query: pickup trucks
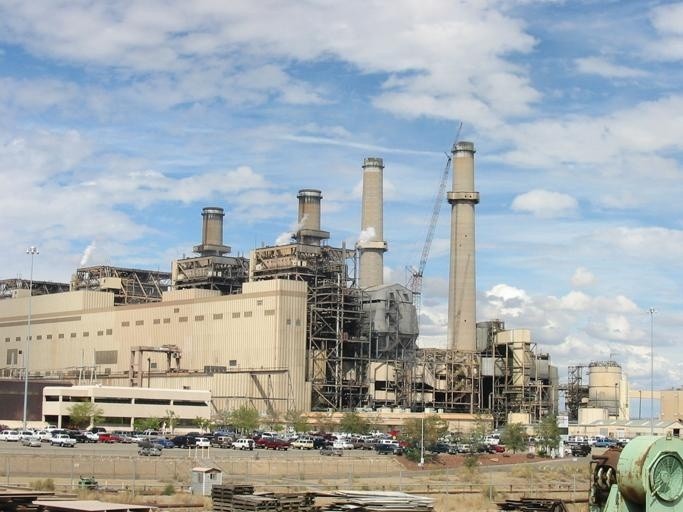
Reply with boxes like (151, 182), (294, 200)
(49, 434), (77, 449)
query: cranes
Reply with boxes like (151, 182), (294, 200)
(406, 119), (464, 349)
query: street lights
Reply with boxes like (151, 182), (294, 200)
(637, 389), (641, 420)
(417, 362), (427, 467)
(146, 357), (151, 388)
(645, 306), (659, 437)
(614, 382), (619, 420)
(20, 245), (40, 427)
(539, 380), (543, 422)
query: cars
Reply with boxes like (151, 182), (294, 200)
(0, 429), (17, 441)
(37, 430), (51, 440)
(530, 435), (631, 457)
(44, 424), (505, 457)
(20, 436), (41, 447)
(18, 430), (36, 438)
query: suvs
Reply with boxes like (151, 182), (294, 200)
(231, 438), (256, 451)
(289, 440), (314, 450)
